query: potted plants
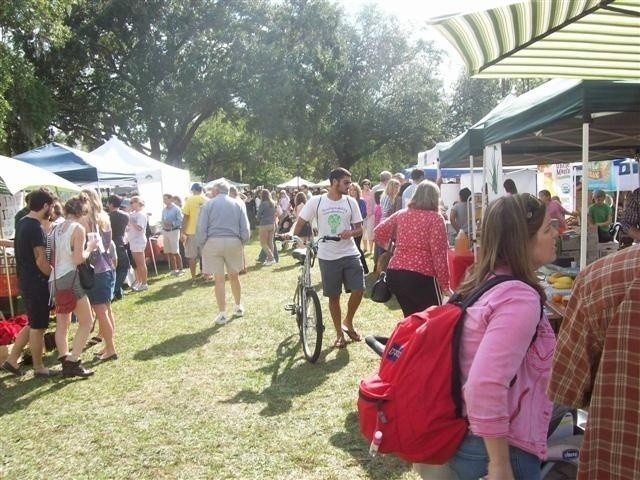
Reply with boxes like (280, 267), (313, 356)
(44, 315), (56, 352)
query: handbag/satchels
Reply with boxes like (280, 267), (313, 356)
(55, 287), (76, 313)
(369, 270), (393, 305)
(79, 262), (97, 289)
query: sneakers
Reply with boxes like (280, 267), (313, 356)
(132, 269), (212, 293)
(232, 302), (244, 316)
(1, 359), (26, 377)
(214, 310), (228, 325)
(31, 369), (58, 380)
(262, 258), (276, 266)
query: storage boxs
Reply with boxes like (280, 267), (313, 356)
(560, 234), (619, 265)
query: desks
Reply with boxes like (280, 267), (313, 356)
(447, 249), (474, 290)
(146, 239), (166, 277)
(541, 285), (569, 328)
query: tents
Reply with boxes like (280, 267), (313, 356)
(276, 174), (331, 187)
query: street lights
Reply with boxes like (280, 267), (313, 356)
(294, 153), (302, 191)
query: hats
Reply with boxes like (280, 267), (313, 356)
(190, 182), (202, 192)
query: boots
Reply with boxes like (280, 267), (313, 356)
(58, 353), (94, 379)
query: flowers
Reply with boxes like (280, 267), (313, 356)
(0, 315), (29, 345)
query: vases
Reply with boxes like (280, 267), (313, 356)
(0, 344), (23, 366)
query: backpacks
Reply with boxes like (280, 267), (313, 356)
(354, 271), (545, 467)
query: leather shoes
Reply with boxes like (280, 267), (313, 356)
(92, 349), (119, 363)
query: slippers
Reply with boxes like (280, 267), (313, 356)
(334, 333), (346, 348)
(342, 323), (362, 341)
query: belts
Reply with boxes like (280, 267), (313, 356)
(162, 225), (180, 232)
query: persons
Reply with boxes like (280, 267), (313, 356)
(4, 188), (118, 377)
(451, 178), (640, 247)
(243, 185), (299, 267)
(362, 169), (453, 319)
(159, 181), (246, 281)
(295, 167), (367, 346)
(105, 194), (148, 298)
(198, 180), (249, 324)
(543, 244), (639, 478)
(450, 194), (557, 480)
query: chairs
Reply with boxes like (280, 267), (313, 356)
(539, 432), (584, 480)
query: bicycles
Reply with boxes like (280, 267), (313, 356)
(270, 232), (343, 362)
